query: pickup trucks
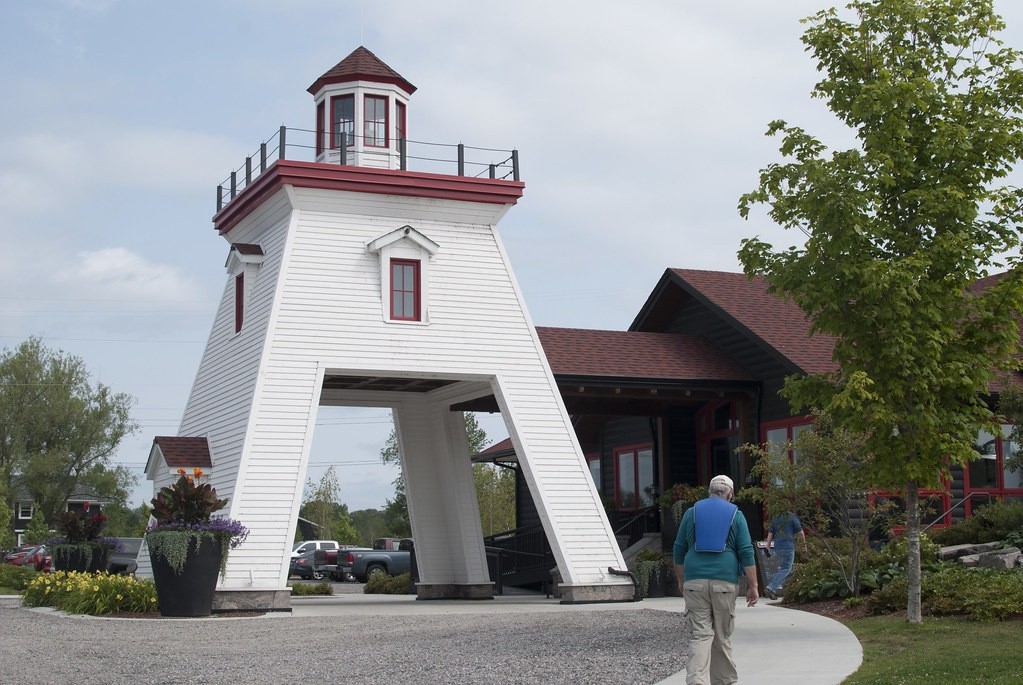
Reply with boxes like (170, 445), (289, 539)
(336, 537), (414, 584)
(315, 537), (402, 583)
(287, 538), (375, 579)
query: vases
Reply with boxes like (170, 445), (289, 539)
(53, 548), (110, 574)
(147, 532), (231, 617)
(648, 565), (668, 598)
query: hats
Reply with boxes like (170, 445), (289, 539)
(710, 475), (735, 502)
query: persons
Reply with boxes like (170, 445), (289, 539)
(672, 475), (759, 685)
(765, 501), (806, 599)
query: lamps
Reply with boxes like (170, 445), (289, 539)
(650, 483), (659, 506)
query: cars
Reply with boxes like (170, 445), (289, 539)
(2, 544), (50, 571)
(291, 549), (341, 581)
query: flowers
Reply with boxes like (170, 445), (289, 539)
(45, 502), (126, 571)
(145, 465), (250, 577)
(657, 482), (763, 529)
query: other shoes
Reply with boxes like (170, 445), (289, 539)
(763, 587), (777, 600)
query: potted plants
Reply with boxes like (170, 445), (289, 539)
(635, 549), (666, 598)
